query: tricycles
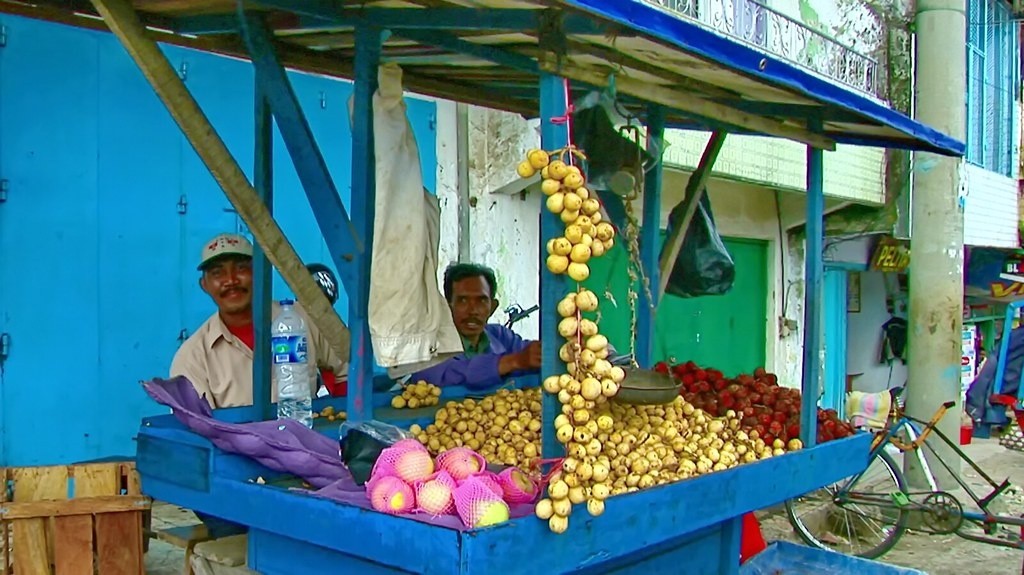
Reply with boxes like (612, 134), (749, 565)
(785, 380), (1024, 562)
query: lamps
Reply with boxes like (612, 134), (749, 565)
(610, 97), (645, 201)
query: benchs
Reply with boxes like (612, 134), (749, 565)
(157, 524), (212, 575)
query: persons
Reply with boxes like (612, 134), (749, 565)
(403, 262), (542, 391)
(169, 234), (348, 538)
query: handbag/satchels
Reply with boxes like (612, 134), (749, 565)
(662, 171), (735, 298)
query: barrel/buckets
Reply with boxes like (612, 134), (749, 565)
(961, 427), (973, 445)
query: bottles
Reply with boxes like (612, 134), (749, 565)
(271, 298), (313, 432)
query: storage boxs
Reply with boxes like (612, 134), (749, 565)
(0, 461), (152, 575)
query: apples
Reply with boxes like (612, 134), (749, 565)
(372, 448), (534, 527)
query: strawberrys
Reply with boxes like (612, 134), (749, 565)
(648, 360), (855, 447)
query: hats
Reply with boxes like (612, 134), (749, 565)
(196, 232), (254, 270)
